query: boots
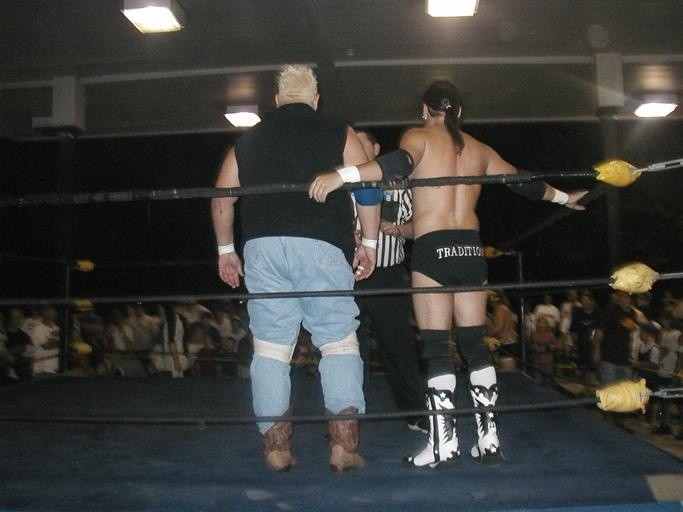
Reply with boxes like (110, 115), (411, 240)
(323, 406), (368, 473)
(403, 387), (462, 467)
(259, 405), (298, 473)
(468, 381), (506, 465)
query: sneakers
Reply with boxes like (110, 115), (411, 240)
(407, 415), (430, 434)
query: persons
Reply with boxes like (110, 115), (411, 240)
(3, 301), (321, 382)
(452, 286), (683, 443)
(208, 65), (385, 474)
(350, 129), (432, 434)
(307, 79), (590, 468)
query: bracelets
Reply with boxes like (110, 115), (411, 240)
(551, 188), (570, 205)
(360, 236), (378, 249)
(337, 166), (362, 185)
(217, 241), (236, 257)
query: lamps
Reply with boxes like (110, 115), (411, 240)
(425, 0), (480, 19)
(223, 105), (264, 129)
(123, 0), (187, 36)
(631, 94), (679, 119)
